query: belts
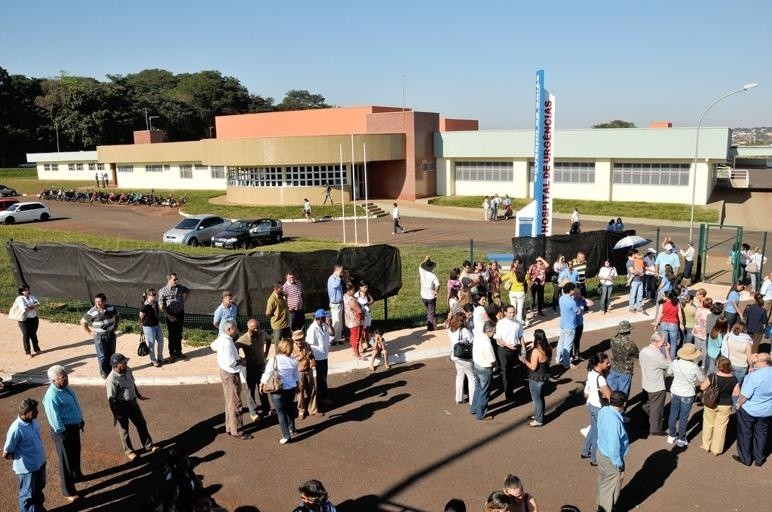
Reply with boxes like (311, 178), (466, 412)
(333, 302), (342, 304)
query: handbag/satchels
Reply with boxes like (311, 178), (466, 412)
(598, 385), (612, 406)
(597, 284), (603, 294)
(138, 340), (148, 356)
(455, 342), (473, 358)
(745, 262), (758, 273)
(8, 304), (27, 322)
(262, 374), (282, 393)
(504, 279), (513, 290)
(701, 385), (719, 409)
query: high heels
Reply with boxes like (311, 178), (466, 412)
(457, 400), (466, 405)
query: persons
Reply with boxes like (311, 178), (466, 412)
(483, 192), (513, 221)
(138, 287), (173, 366)
(606, 219), (616, 230)
(2, 397), (50, 512)
(158, 271), (191, 363)
(320, 182), (334, 206)
(94, 172), (110, 188)
(390, 202), (407, 235)
(10, 279), (48, 358)
(208, 263), (392, 446)
(42, 364), (92, 505)
(301, 198), (314, 224)
(417, 233), (772, 512)
(614, 217), (625, 231)
(79, 292), (121, 379)
(105, 352), (163, 461)
(235, 478), (339, 512)
(566, 205), (583, 235)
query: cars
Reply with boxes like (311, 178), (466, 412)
(0, 199), (51, 224)
(0, 185), (18, 197)
(162, 214), (232, 249)
(0, 198), (19, 211)
(209, 215), (283, 248)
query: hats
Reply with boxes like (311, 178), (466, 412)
(421, 258), (436, 271)
(314, 309), (331, 318)
(677, 343), (701, 360)
(648, 247), (657, 253)
(616, 321), (633, 333)
(111, 353), (130, 364)
(292, 330), (304, 340)
(665, 243), (673, 250)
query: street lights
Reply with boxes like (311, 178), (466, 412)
(688, 82), (759, 245)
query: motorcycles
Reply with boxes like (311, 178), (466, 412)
(39, 187), (177, 208)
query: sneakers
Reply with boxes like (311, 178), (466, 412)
(624, 416), (630, 423)
(226, 409), (325, 445)
(67, 494), (80, 501)
(485, 415), (493, 419)
(652, 431), (689, 447)
(128, 452), (137, 460)
(322, 398), (333, 404)
(529, 421), (542, 426)
(153, 354), (187, 366)
(151, 446), (161, 453)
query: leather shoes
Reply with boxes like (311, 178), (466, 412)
(732, 455), (742, 462)
